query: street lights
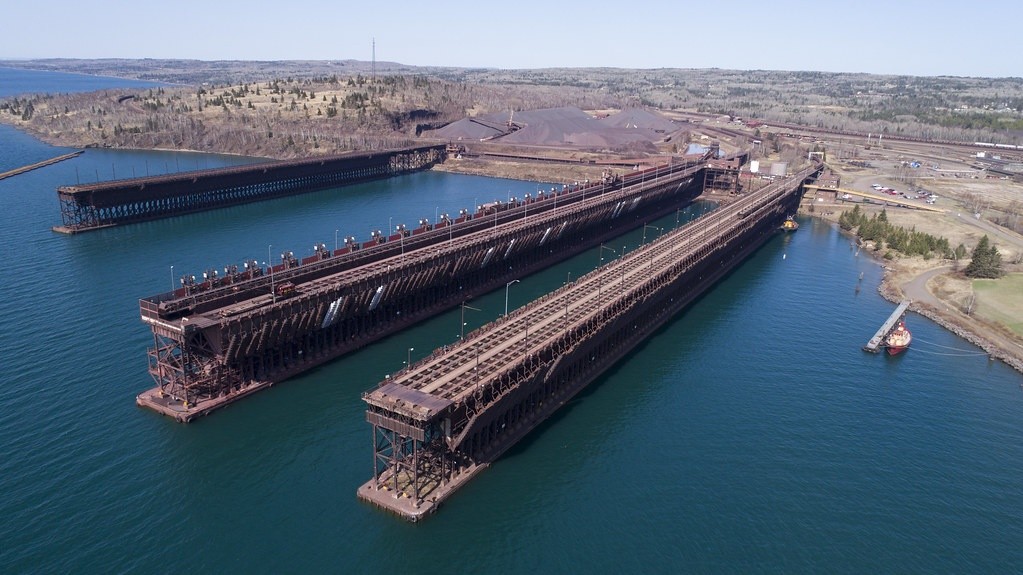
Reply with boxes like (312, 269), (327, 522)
(518, 198), (526, 224)
(389, 217), (393, 236)
(262, 262), (275, 305)
(408, 347), (414, 363)
(269, 245), (272, 266)
(171, 266), (174, 291)
(599, 242), (615, 268)
(677, 207), (686, 227)
(505, 279), (520, 316)
(548, 190), (556, 216)
(335, 229), (339, 250)
(435, 206), (439, 226)
(642, 222), (659, 246)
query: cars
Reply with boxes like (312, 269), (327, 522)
(871, 183), (938, 203)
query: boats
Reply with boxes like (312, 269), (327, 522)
(780, 215), (799, 232)
(885, 321), (913, 356)
